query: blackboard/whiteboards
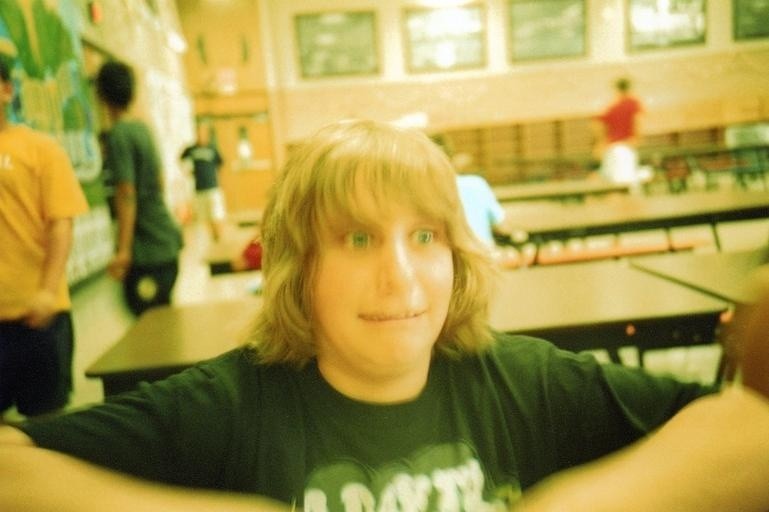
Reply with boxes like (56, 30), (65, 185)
(0, 0), (119, 296)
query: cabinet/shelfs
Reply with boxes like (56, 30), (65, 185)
(449, 111), (609, 180)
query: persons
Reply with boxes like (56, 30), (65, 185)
(429, 130), (508, 266)
(594, 79), (648, 179)
(0, 52), (92, 422)
(0, 117), (769, 511)
(179, 121), (227, 241)
(93, 62), (187, 315)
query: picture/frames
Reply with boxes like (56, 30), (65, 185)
(291, 1), (769, 82)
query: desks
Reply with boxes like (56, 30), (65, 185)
(472, 138), (768, 386)
(85, 210), (266, 402)
